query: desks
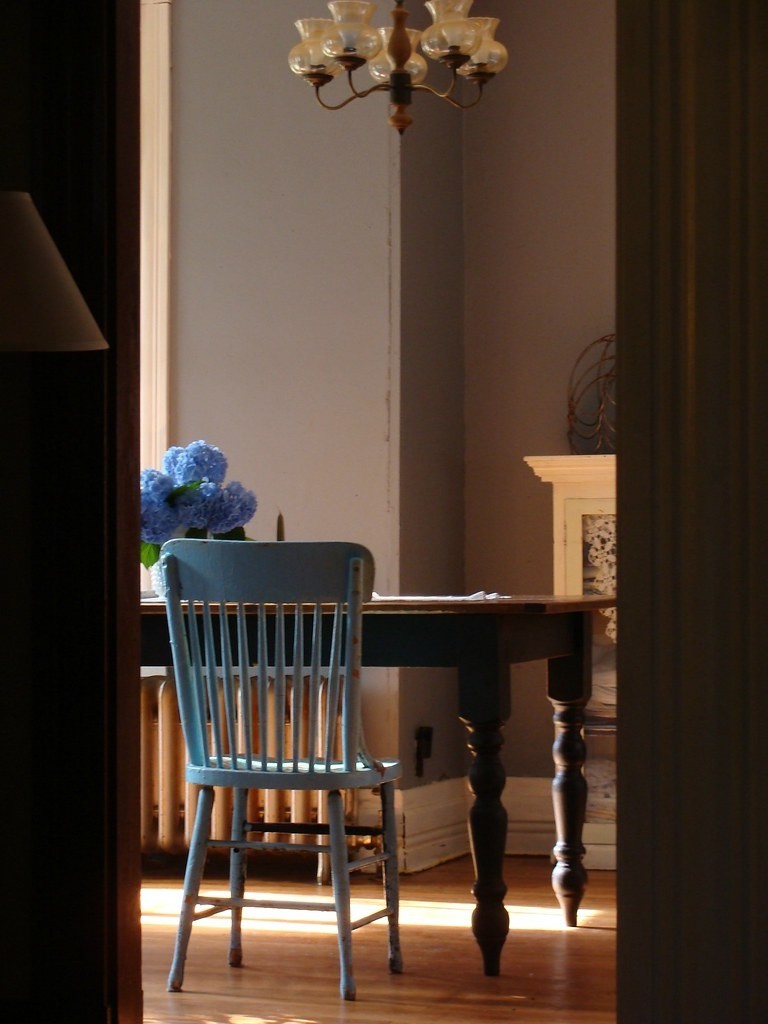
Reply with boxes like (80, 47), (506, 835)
(141, 596), (617, 975)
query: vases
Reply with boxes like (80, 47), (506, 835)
(150, 523), (214, 598)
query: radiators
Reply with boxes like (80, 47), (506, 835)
(141, 675), (352, 886)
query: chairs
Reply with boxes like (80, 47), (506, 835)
(159, 538), (402, 1000)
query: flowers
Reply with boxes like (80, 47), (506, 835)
(139, 440), (257, 568)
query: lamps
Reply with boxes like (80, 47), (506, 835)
(288, 0), (509, 135)
(1, 189), (109, 352)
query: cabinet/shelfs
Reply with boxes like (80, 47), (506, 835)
(523, 454), (616, 869)
